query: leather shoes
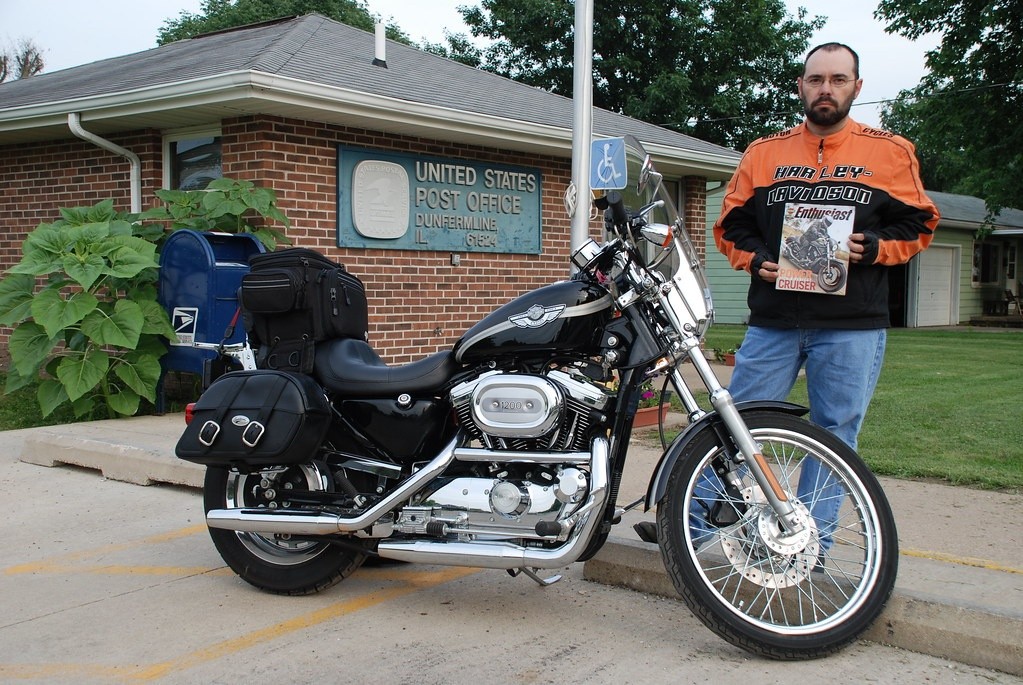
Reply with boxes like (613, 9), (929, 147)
(812, 555), (825, 574)
(633, 520), (699, 551)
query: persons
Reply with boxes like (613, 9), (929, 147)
(799, 216), (834, 257)
(633, 41), (940, 575)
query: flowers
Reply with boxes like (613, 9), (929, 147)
(610, 376), (663, 409)
(728, 344), (741, 354)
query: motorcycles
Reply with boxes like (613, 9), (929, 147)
(782, 229), (846, 293)
(174, 133), (900, 663)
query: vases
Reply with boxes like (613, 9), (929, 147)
(631, 402), (670, 429)
(725, 353), (736, 366)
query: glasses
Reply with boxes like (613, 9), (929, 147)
(803, 77), (855, 87)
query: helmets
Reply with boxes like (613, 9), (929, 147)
(822, 214), (833, 228)
